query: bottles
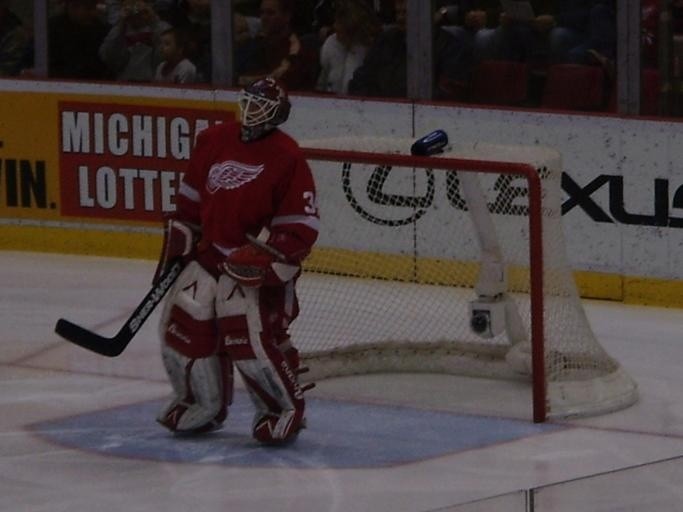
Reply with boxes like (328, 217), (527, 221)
(411, 129), (450, 155)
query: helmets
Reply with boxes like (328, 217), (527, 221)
(238, 77), (290, 143)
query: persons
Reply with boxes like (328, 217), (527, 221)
(0, 0), (683, 120)
(150, 75), (319, 441)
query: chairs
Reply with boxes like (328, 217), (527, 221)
(474, 58), (662, 115)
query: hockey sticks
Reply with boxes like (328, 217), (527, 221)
(54, 261), (184, 357)
